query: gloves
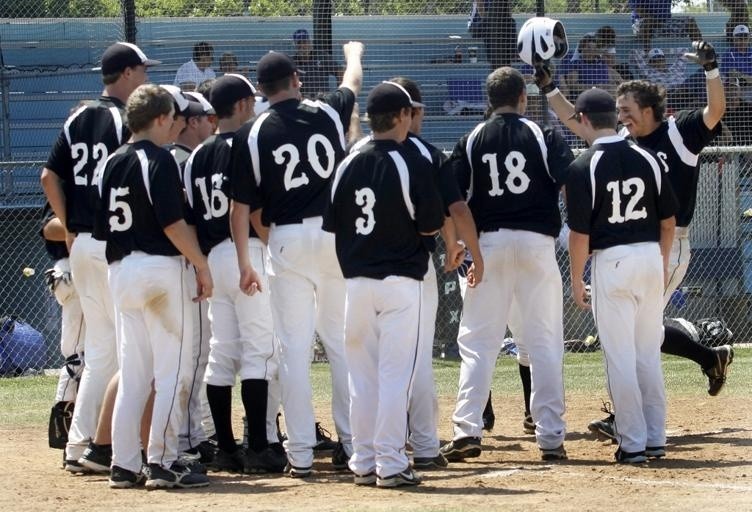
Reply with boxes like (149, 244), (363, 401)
(44, 269), (73, 291)
(684, 40), (718, 71)
(531, 61), (556, 94)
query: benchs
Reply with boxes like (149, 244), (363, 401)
(0, 12), (751, 205)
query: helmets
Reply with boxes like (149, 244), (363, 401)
(517, 16), (569, 65)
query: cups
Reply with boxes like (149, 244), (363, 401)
(741, 208), (752, 225)
(468, 47), (478, 63)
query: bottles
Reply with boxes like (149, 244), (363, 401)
(23, 267), (35, 277)
(631, 20), (640, 35)
(677, 286), (704, 298)
(455, 44), (462, 63)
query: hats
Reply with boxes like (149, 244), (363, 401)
(293, 30), (308, 42)
(647, 48), (664, 62)
(102, 41), (163, 74)
(257, 49), (307, 82)
(366, 80), (427, 113)
(568, 86), (616, 121)
(210, 73), (257, 102)
(159, 84), (216, 116)
(733, 24), (749, 36)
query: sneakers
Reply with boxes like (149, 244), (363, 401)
(615, 446), (665, 463)
(540, 445), (567, 460)
(481, 415), (494, 429)
(441, 439), (482, 461)
(705, 344), (734, 396)
(314, 421), (339, 451)
(413, 450), (449, 469)
(524, 412), (537, 435)
(588, 414), (615, 440)
(188, 433), (313, 477)
(333, 443), (422, 488)
(66, 440), (209, 488)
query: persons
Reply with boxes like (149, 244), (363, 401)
(40, 0), (751, 490)
